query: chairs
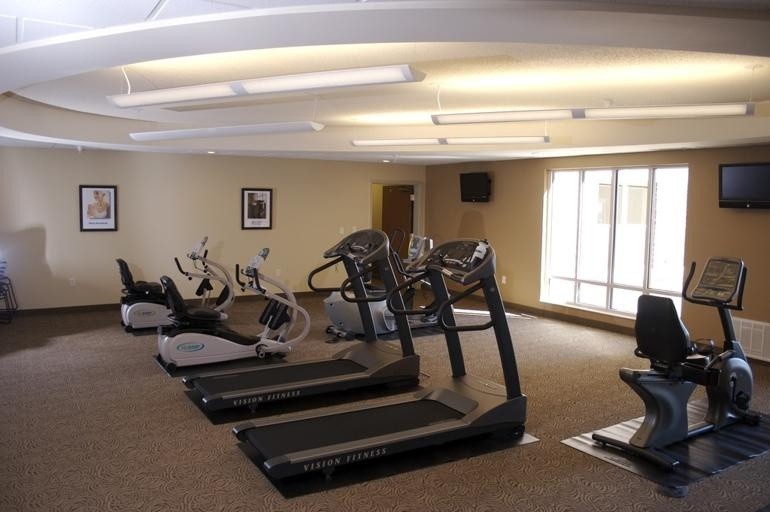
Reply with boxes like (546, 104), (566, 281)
(634, 295), (716, 380)
(160, 276), (221, 329)
(116, 258), (163, 296)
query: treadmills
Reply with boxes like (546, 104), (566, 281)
(182, 230), (420, 412)
(234, 239), (526, 478)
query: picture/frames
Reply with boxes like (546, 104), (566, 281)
(241, 187), (272, 231)
(79, 184), (117, 233)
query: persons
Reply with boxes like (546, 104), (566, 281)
(87, 191), (111, 219)
(248, 194), (259, 218)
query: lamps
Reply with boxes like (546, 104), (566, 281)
(344, 115), (551, 149)
(428, 66), (761, 126)
(103, 57), (421, 108)
(129, 96), (326, 144)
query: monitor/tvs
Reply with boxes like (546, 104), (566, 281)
(719, 162), (770, 209)
(460, 172), (489, 202)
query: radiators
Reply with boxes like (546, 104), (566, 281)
(728, 314), (770, 363)
